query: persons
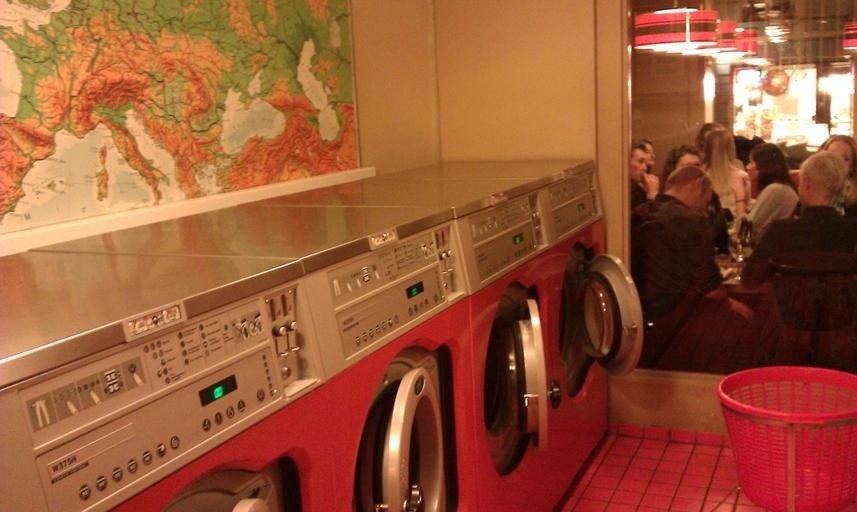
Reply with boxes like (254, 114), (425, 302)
(630, 123), (856, 327)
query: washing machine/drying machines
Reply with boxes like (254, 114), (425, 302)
(238, 179), (566, 512)
(0, 249), (337, 512)
(27, 205), (478, 512)
(361, 159), (645, 502)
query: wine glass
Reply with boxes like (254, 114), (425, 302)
(729, 231), (748, 283)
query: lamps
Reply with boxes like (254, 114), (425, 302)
(843, 21), (857, 50)
(633, 0), (759, 56)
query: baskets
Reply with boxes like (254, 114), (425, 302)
(716, 365), (857, 512)
